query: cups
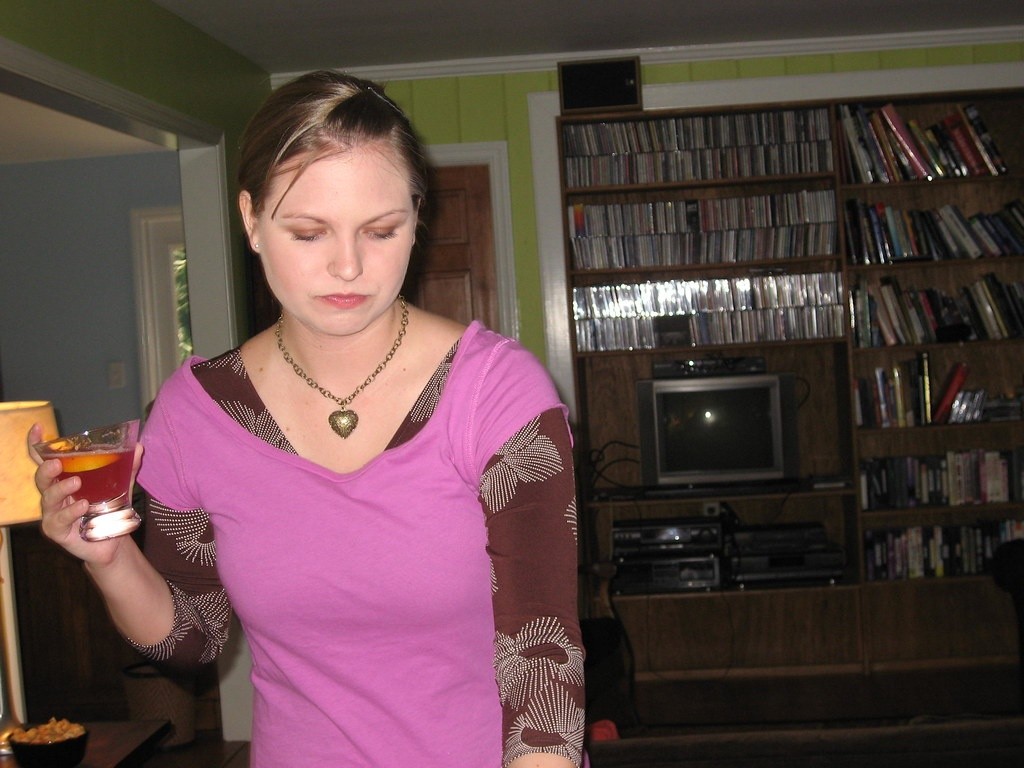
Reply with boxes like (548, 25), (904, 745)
(32, 417), (140, 540)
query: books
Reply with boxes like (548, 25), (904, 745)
(570, 273), (1024, 352)
(853, 349), (1024, 426)
(564, 103), (1024, 182)
(858, 440), (1024, 512)
(564, 191), (1024, 265)
(862, 515), (1024, 586)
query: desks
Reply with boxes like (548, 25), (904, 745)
(0, 719), (173, 768)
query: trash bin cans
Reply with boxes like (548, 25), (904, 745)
(119, 661), (198, 749)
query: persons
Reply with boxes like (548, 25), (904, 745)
(28, 69), (585, 768)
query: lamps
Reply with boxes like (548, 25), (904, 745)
(0, 400), (61, 726)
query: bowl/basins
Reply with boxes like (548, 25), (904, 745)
(7, 725), (89, 768)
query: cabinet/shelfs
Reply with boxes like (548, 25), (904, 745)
(551, 87), (1024, 768)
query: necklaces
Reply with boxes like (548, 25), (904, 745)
(275, 293), (409, 441)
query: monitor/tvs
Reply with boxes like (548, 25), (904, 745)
(637, 373), (786, 498)
